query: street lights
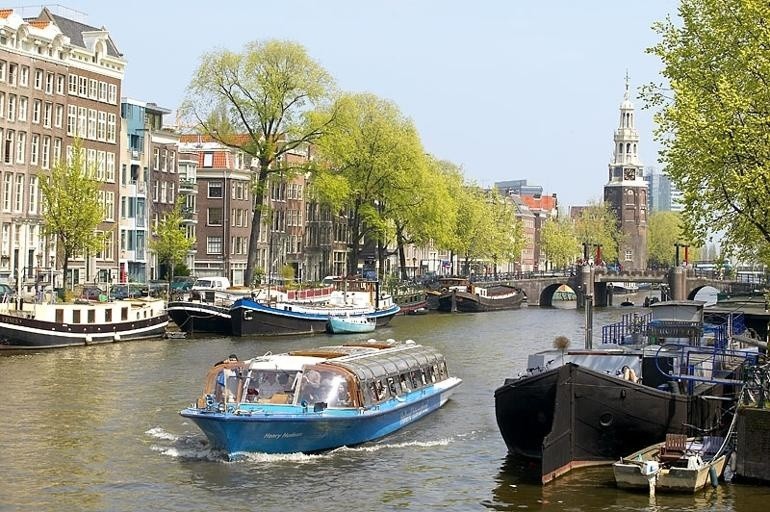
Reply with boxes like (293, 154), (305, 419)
(412, 257), (416, 280)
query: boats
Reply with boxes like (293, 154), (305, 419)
(1, 296), (169, 356)
(167, 276), (401, 336)
(439, 279), (524, 310)
(396, 294), (429, 314)
(179, 339), (462, 462)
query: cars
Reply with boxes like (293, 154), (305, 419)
(1, 285), (15, 302)
(82, 284), (166, 300)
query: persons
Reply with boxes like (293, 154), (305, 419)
(618, 366), (639, 382)
(303, 370), (444, 406)
(570, 258), (624, 277)
